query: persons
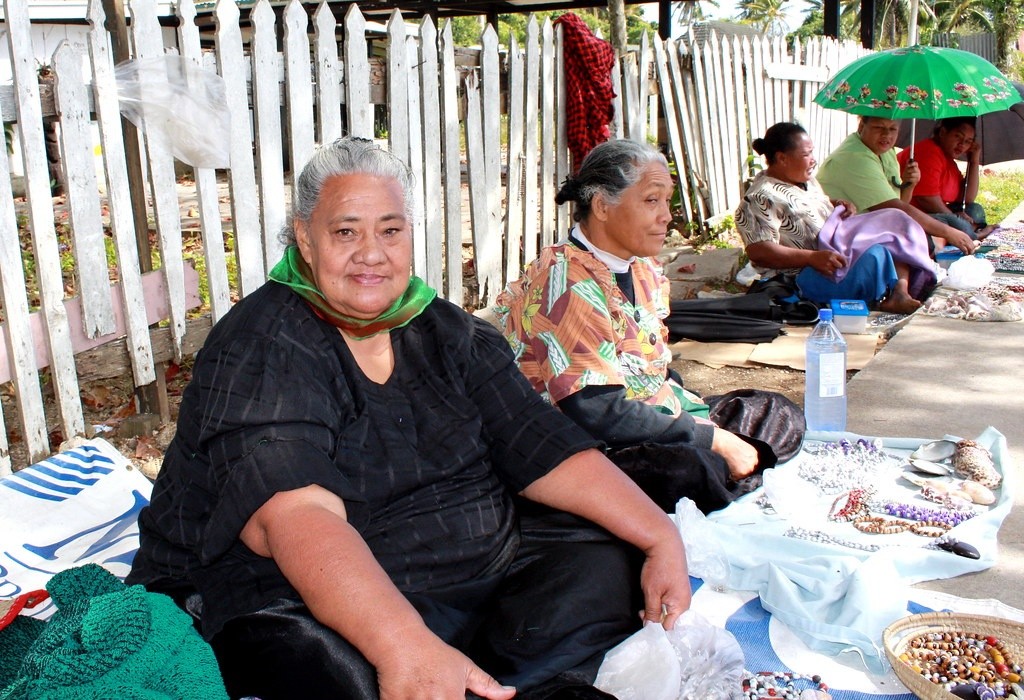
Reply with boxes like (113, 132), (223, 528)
(124, 136), (692, 700)
(497, 139), (807, 514)
(896, 116), (1001, 240)
(815, 117), (975, 256)
(734, 122), (924, 313)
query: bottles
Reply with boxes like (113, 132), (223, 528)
(803, 308), (848, 432)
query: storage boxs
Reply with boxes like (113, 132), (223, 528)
(829, 299), (870, 333)
(936, 246), (963, 269)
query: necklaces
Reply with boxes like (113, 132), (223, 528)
(742, 671), (832, 700)
(754, 438), (981, 561)
(898, 631), (1024, 700)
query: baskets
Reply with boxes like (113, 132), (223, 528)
(883, 611), (1024, 700)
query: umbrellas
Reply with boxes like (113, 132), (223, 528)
(811, 43), (1024, 213)
